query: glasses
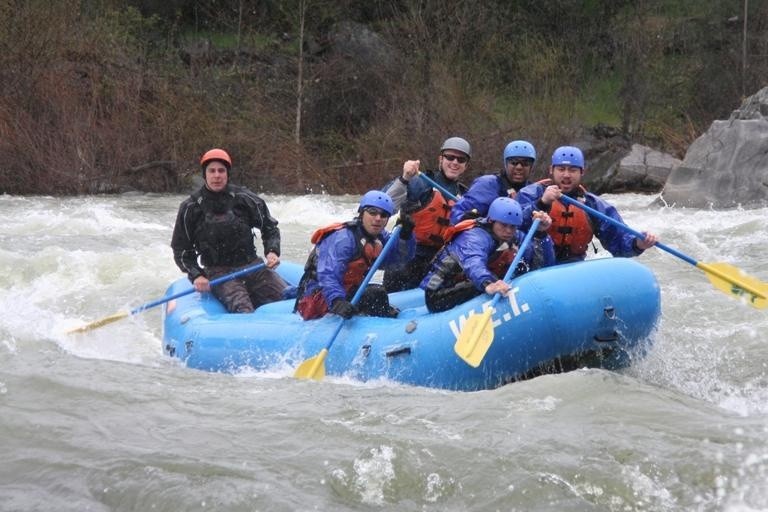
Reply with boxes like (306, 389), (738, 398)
(442, 154), (467, 163)
(507, 158), (532, 167)
(363, 208), (387, 218)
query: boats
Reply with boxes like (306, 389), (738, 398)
(160, 254), (662, 395)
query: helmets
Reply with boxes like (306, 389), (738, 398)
(359, 190), (394, 215)
(200, 149), (232, 167)
(488, 197), (523, 226)
(503, 140), (536, 169)
(441, 137), (472, 157)
(552, 146), (584, 168)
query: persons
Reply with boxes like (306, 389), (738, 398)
(171, 148), (291, 314)
(380, 137), (470, 283)
(294, 189), (398, 321)
(512, 146), (658, 265)
(448, 141), (538, 226)
(421, 198), (525, 312)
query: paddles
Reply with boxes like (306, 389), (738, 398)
(454, 217), (542, 368)
(58, 262), (270, 338)
(294, 223), (403, 381)
(558, 193), (768, 308)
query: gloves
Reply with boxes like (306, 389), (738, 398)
(396, 215), (415, 240)
(332, 297), (359, 320)
